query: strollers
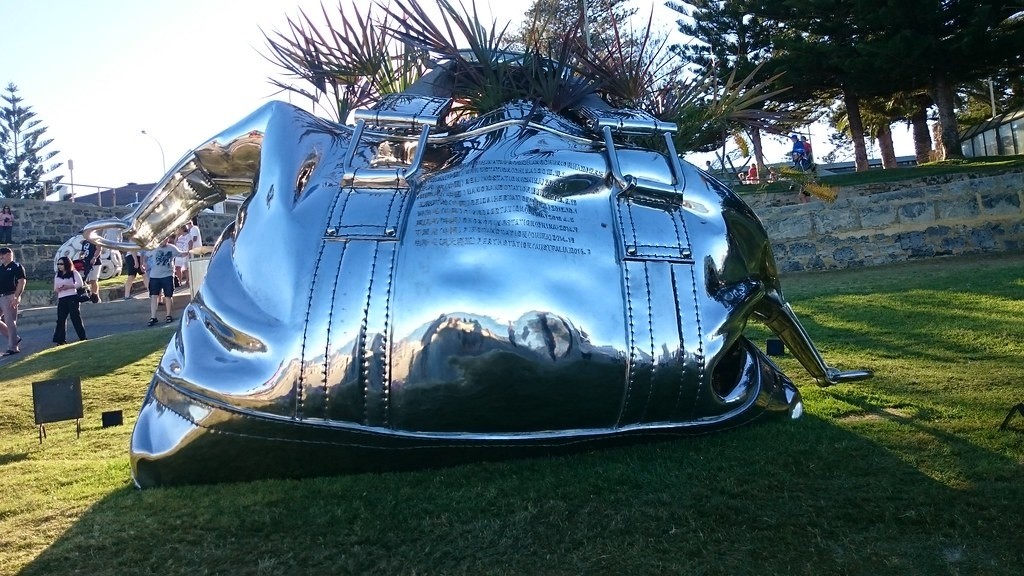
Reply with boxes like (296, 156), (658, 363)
(72, 259), (99, 304)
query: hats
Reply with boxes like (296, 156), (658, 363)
(78, 228), (84, 233)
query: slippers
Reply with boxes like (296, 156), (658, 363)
(3, 349), (21, 356)
(16, 338), (21, 345)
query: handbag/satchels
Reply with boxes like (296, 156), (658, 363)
(77, 287), (90, 302)
(130, 53), (874, 491)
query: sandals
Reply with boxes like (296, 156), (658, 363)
(148, 317), (157, 327)
(166, 316), (173, 323)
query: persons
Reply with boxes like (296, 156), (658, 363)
(706, 161), (713, 172)
(124, 239), (149, 300)
(140, 221), (202, 326)
(0, 206), (14, 245)
(0, 248), (26, 356)
(791, 135), (817, 203)
(769, 166), (778, 182)
(78, 228), (102, 303)
(421, 314), (573, 361)
(53, 257), (86, 346)
(747, 164), (758, 184)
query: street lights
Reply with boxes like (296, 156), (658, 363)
(142, 130), (165, 175)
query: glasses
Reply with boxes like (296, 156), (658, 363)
(4, 208), (9, 209)
(57, 263), (65, 265)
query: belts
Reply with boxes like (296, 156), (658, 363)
(0, 292), (15, 297)
